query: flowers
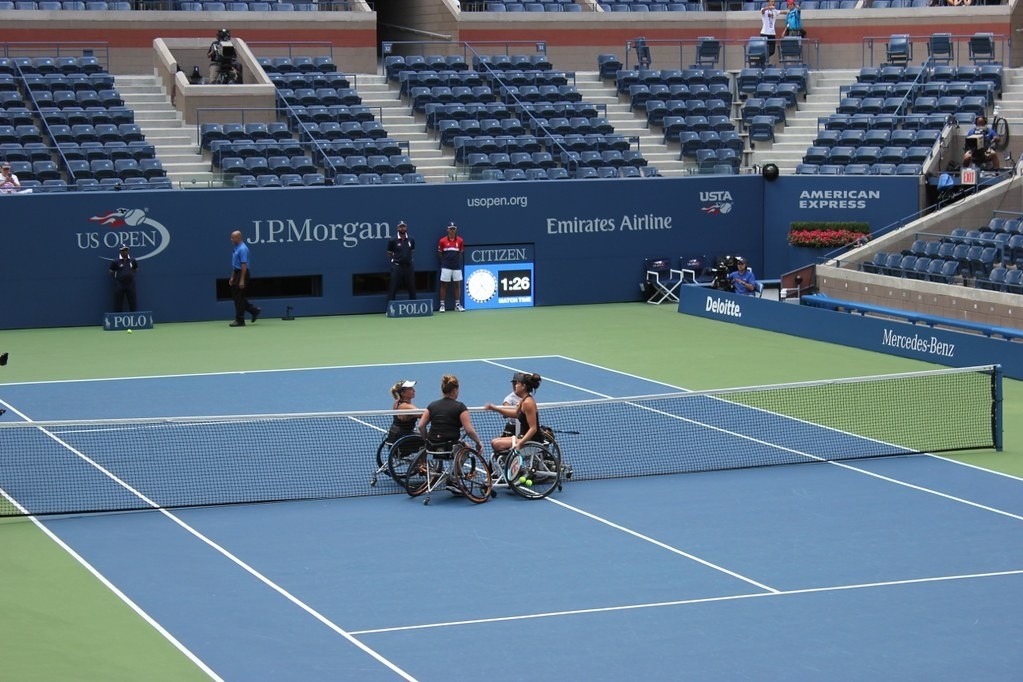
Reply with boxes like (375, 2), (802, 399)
(787, 228), (869, 243)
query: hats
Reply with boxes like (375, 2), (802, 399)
(510, 373), (533, 384)
(401, 380), (417, 388)
(738, 257), (746, 264)
(787, 0), (794, 4)
(1, 162), (11, 168)
(120, 244), (129, 251)
(447, 222), (457, 229)
(397, 220), (407, 226)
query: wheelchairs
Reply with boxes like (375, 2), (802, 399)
(376, 430), (443, 492)
(404, 441), (498, 504)
(481, 431), (564, 501)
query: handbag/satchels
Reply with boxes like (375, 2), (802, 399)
(797, 29), (806, 38)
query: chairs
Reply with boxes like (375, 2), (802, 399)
(755, 282), (765, 298)
(197, 32), (1004, 189)
(0, 0), (319, 12)
(482, 0), (940, 13)
(644, 257), (719, 306)
(863, 215), (1023, 294)
(0, 56), (173, 194)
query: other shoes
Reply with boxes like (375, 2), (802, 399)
(229, 320), (245, 327)
(251, 309), (260, 322)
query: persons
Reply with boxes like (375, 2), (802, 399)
(207, 34), (238, 84)
(228, 230), (262, 327)
(109, 243), (139, 314)
(384, 372), (482, 493)
(725, 257), (756, 300)
(0, 161), (20, 192)
(484, 372), (542, 466)
(759, 0), (802, 65)
(438, 221), (466, 313)
(961, 116), (1001, 170)
(386, 220), (417, 316)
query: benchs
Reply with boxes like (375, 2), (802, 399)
(801, 294), (1023, 345)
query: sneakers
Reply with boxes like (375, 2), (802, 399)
(455, 304), (465, 312)
(446, 478), (463, 493)
(439, 302), (445, 313)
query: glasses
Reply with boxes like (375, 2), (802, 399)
(739, 263), (743, 265)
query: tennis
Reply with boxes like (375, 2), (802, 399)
(519, 476), (526, 483)
(525, 479), (533, 487)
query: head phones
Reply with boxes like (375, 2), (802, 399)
(974, 116), (988, 125)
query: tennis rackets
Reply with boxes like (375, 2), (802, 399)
(381, 427), (417, 442)
(504, 435), (524, 483)
(551, 430), (579, 434)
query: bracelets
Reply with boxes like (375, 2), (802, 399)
(475, 441), (481, 445)
